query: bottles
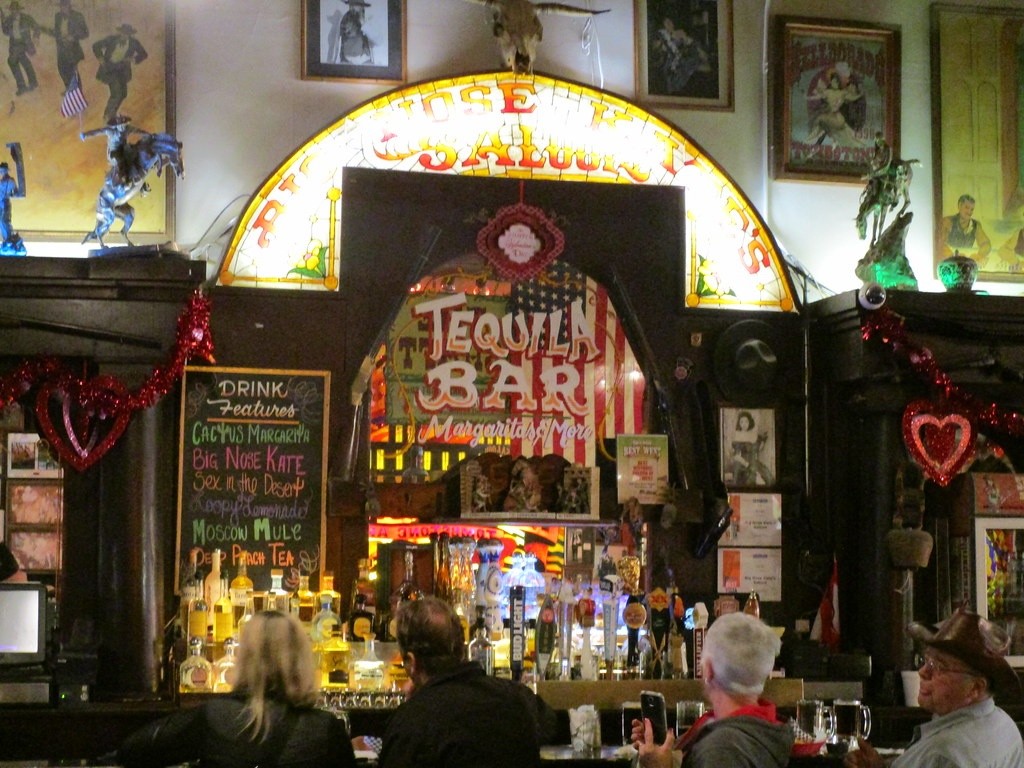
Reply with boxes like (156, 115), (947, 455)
(213, 638), (238, 693)
(270, 569), (289, 615)
(321, 624), (350, 692)
(179, 638), (213, 693)
(349, 595), (373, 642)
(180, 550), (276, 664)
(403, 445), (430, 484)
(386, 552), (424, 641)
(354, 634), (385, 693)
(390, 653), (413, 692)
(449, 591), (497, 677)
(291, 576), (343, 669)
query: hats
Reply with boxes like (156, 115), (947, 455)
(906, 607), (1021, 705)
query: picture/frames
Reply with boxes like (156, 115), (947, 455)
(717, 398), (783, 493)
(930, 3), (1024, 284)
(0, 0), (176, 247)
(775, 13), (903, 188)
(968, 512), (1024, 670)
(4, 429), (68, 482)
(301, 0), (407, 88)
(632, 0), (736, 114)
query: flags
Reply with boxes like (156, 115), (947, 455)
(61, 72), (88, 118)
(509, 260), (647, 468)
(809, 560), (841, 654)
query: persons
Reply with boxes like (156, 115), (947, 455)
(730, 411), (775, 488)
(472, 475), (590, 514)
(842, 608), (1024, 768)
(870, 131), (899, 208)
(80, 114), (157, 197)
(0, 542), (23, 583)
(630, 612), (796, 768)
(113, 612), (355, 768)
(375, 596), (542, 768)
(0, 163), (18, 249)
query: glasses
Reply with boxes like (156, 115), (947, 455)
(914, 654), (981, 678)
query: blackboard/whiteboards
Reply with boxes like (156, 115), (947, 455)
(174, 362), (332, 598)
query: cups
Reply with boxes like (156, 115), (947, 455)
(796, 701), (835, 755)
(833, 701), (871, 752)
(622, 703), (642, 746)
(676, 702), (704, 737)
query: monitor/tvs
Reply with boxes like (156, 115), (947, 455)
(0, 582), (48, 665)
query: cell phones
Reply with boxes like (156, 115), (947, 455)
(641, 691), (668, 747)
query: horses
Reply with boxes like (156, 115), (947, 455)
(82, 132), (184, 249)
(852, 157), (922, 248)
